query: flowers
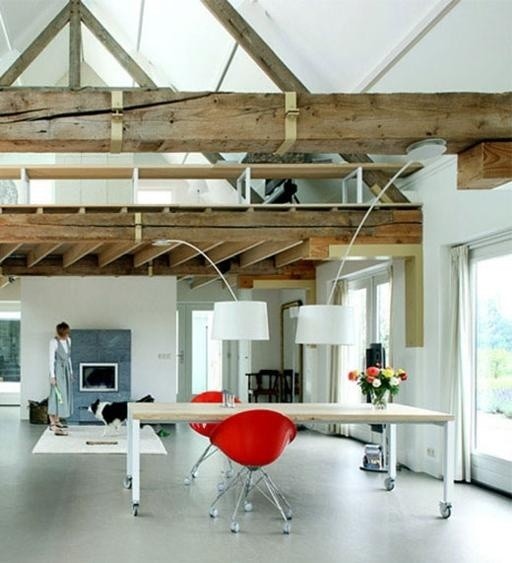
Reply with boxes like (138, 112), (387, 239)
(349, 367), (408, 409)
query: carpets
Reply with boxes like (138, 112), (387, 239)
(31, 423), (168, 455)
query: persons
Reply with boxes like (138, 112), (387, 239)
(47, 322), (75, 432)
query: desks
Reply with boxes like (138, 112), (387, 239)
(117, 397), (459, 525)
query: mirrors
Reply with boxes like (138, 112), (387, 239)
(279, 299), (302, 403)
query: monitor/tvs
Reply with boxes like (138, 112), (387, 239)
(371, 343), (385, 369)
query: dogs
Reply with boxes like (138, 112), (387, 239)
(88, 394), (154, 437)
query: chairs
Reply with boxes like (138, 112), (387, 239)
(244, 367), (281, 403)
(185, 390), (245, 491)
(207, 409), (298, 536)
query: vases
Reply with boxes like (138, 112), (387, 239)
(373, 400), (387, 413)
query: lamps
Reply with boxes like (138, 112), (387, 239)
(294, 138), (448, 347)
(154, 234), (272, 342)
